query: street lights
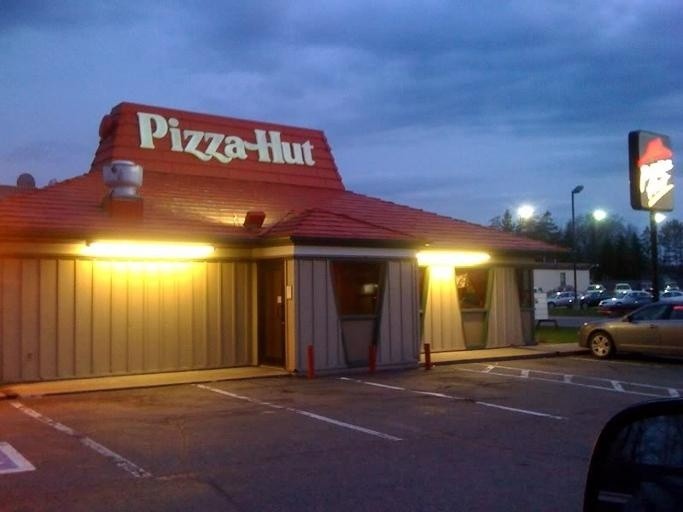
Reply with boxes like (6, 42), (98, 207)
(572, 185), (584, 303)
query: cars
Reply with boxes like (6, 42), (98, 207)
(641, 281), (653, 292)
(599, 291), (652, 307)
(586, 283), (605, 294)
(660, 280), (683, 303)
(547, 290), (581, 309)
(576, 297), (683, 360)
(615, 282), (632, 294)
